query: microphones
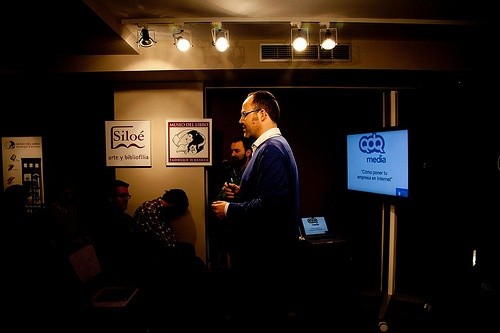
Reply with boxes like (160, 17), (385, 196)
(223, 160), (234, 190)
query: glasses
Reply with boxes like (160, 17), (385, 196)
(241, 109), (267, 119)
(117, 194), (131, 199)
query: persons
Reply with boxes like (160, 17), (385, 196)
(134, 189), (189, 249)
(53, 175), (133, 248)
(217, 137), (253, 203)
(211, 90), (299, 280)
(4, 185), (29, 215)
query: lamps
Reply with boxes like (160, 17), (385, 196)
(170, 23), (194, 52)
(318, 21), (339, 51)
(210, 22), (230, 52)
(135, 23), (158, 49)
(290, 22), (310, 52)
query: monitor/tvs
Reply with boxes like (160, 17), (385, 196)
(345, 125), (416, 202)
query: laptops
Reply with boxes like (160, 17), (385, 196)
(300, 216), (341, 240)
